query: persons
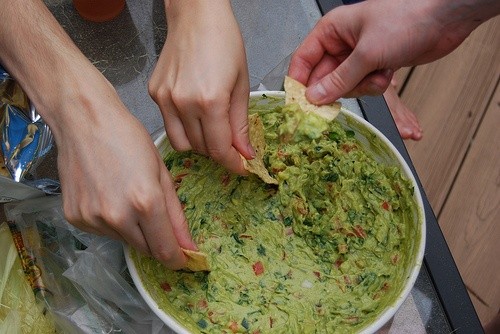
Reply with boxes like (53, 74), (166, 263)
(382, 73), (422, 140)
(147, 0), (256, 175)
(287, 1), (499, 106)
(0, 0), (200, 271)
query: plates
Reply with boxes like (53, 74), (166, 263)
(123, 91), (426, 333)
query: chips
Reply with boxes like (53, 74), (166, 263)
(0, 71), (55, 182)
(239, 112), (277, 182)
(181, 247), (209, 271)
(283, 75), (342, 135)
